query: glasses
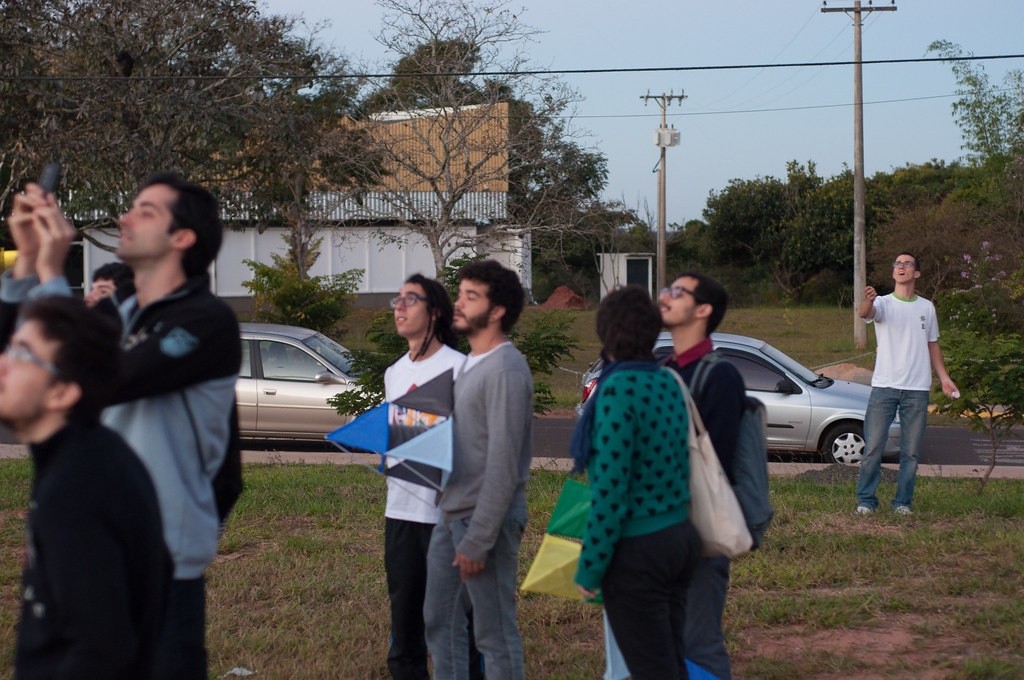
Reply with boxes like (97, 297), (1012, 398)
(660, 287), (695, 300)
(892, 262), (915, 268)
(390, 292), (426, 309)
(5, 343), (59, 379)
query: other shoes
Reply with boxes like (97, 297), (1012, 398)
(894, 506), (911, 514)
(857, 505), (872, 514)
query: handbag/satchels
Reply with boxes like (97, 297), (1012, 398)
(667, 367), (753, 558)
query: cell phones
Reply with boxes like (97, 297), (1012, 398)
(39, 163), (61, 197)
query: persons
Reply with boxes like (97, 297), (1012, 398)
(572, 287), (695, 680)
(422, 260), (534, 680)
(382, 273), (470, 680)
(856, 252), (960, 517)
(0, 173), (243, 680)
(652, 268), (745, 679)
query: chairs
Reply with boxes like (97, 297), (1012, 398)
(269, 343), (289, 377)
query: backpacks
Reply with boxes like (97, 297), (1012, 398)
(167, 300), (242, 524)
(690, 355), (774, 552)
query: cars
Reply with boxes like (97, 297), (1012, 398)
(576, 334), (902, 466)
(234, 322), (386, 442)
